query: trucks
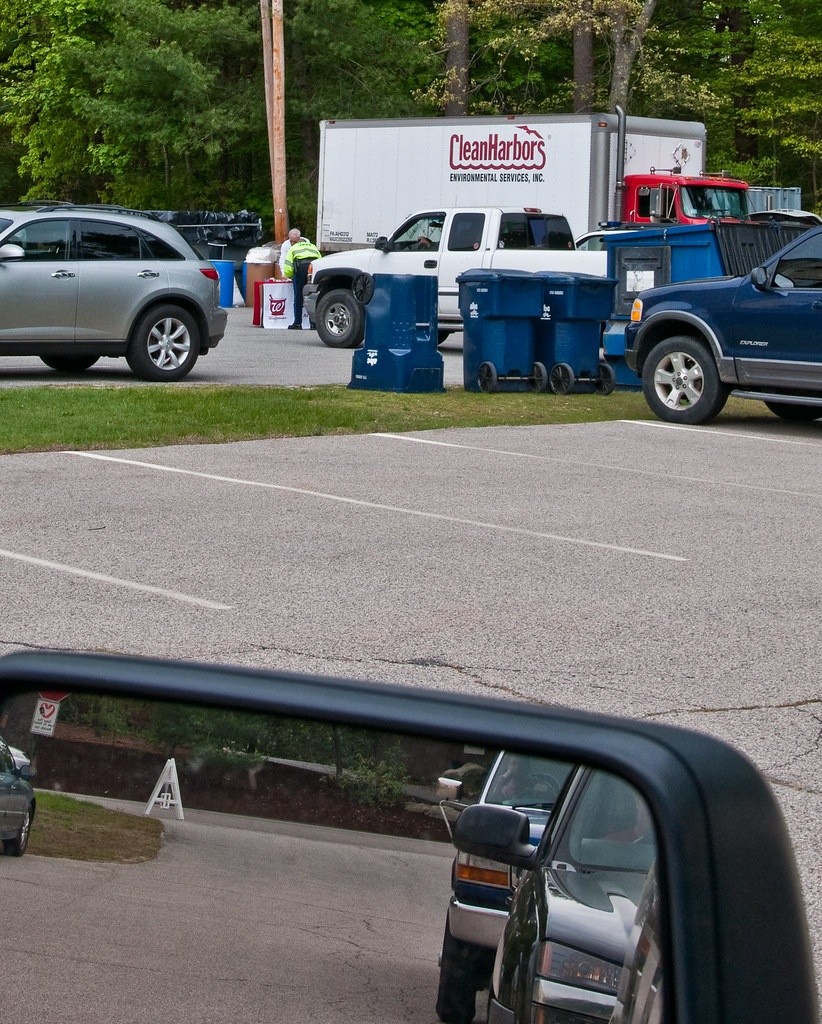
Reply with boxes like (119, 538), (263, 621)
(311, 105), (751, 249)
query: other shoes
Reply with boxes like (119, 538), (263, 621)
(309, 323), (315, 328)
(287, 323), (302, 331)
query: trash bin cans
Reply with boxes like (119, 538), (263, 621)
(346, 270), (448, 392)
(537, 270), (618, 394)
(602, 218), (822, 390)
(242, 260), (282, 308)
(206, 260), (236, 308)
(457, 268), (549, 394)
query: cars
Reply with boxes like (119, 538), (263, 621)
(436, 751), (581, 1022)
(0, 196), (230, 380)
(449, 755), (655, 1024)
(0, 736), (39, 856)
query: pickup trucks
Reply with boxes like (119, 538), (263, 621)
(301, 205), (611, 350)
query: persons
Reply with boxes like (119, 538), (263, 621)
(601, 787), (652, 845)
(279, 229), (322, 330)
(486, 749), (553, 807)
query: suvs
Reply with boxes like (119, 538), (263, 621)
(619, 224), (822, 424)
